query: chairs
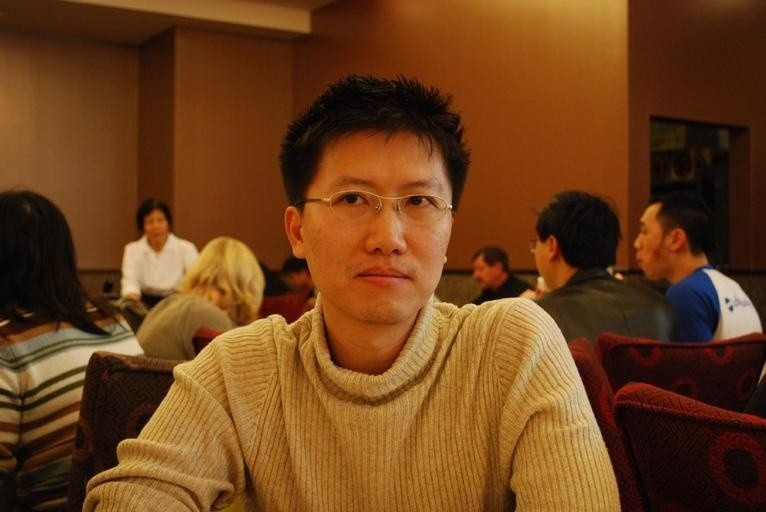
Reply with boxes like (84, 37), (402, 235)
(67, 351), (192, 512)
(192, 325), (225, 355)
(594, 331), (766, 417)
(611, 381), (766, 511)
(258, 291), (312, 324)
(567, 336), (646, 511)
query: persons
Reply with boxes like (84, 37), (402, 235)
(0, 190), (149, 511)
(528, 190), (697, 352)
(471, 246), (530, 305)
(634, 192), (763, 344)
(120, 199), (319, 360)
(82, 75), (622, 512)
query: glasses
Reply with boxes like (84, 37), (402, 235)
(305, 189), (453, 224)
